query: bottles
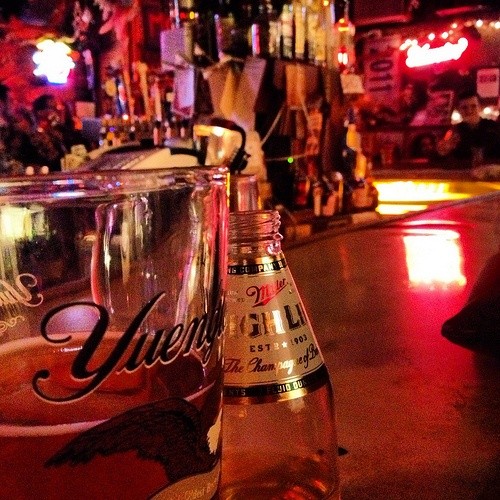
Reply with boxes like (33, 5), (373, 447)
(221, 209), (342, 500)
(180, 1), (306, 64)
(333, 17), (355, 72)
(312, 16), (327, 67)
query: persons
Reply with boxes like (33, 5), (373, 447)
(359, 82), (499, 170)
(0, 71), (145, 284)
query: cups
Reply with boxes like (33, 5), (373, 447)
(0, 166), (230, 500)
